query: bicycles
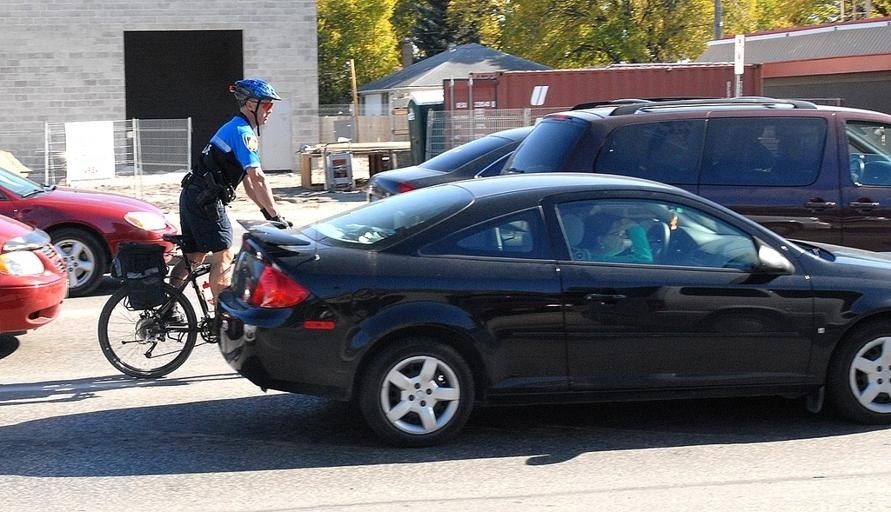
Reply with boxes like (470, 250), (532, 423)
(95, 210), (294, 384)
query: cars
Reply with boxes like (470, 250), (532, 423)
(498, 92), (891, 250)
(219, 171), (891, 456)
(2, 210), (69, 348)
(0, 168), (180, 299)
(366, 124), (531, 213)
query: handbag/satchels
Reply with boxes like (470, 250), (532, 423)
(110, 242), (166, 309)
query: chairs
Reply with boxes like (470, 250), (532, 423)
(559, 212), (593, 261)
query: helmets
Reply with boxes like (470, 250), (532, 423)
(230, 79), (281, 106)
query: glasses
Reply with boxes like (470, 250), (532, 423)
(251, 101), (272, 111)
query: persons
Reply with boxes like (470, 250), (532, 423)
(161, 77), (283, 315)
(587, 210), (655, 267)
